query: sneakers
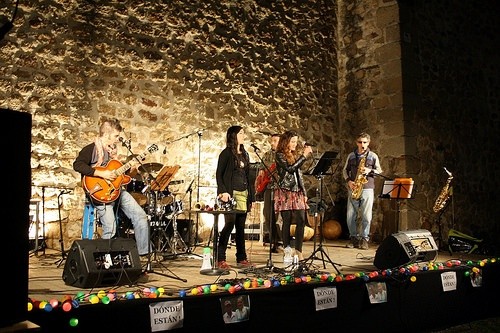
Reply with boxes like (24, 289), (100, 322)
(215, 260), (231, 269)
(237, 260), (254, 267)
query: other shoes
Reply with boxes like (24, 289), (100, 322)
(295, 250), (304, 261)
(346, 241), (358, 248)
(140, 253), (163, 262)
(358, 239), (368, 249)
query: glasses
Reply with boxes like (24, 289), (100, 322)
(358, 141), (366, 144)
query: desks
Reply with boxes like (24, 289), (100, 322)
(187, 210), (247, 275)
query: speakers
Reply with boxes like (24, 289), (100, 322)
(62, 238), (142, 288)
(374, 228), (439, 269)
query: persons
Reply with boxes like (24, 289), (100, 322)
(257, 134), (282, 253)
(343, 130), (382, 250)
(235, 296), (250, 322)
(73, 119), (165, 263)
(216, 126), (256, 270)
(275, 130), (313, 263)
(223, 300), (237, 323)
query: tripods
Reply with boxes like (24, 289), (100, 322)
(143, 125), (212, 282)
(30, 185), (71, 268)
(434, 193), (454, 256)
(254, 149), (342, 277)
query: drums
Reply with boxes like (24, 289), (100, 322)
(156, 186), (174, 206)
(165, 199), (184, 219)
(306, 209), (325, 241)
(125, 175), (149, 206)
(113, 204), (158, 238)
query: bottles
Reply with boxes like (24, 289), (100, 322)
(293, 255), (299, 269)
(283, 244), (293, 272)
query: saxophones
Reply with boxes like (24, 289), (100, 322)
(432, 167), (453, 213)
(351, 147), (369, 201)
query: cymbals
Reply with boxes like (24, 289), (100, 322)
(137, 162), (164, 172)
(126, 154), (145, 162)
(171, 192), (185, 196)
(168, 179), (185, 185)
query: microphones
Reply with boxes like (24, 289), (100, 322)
(163, 138), (169, 154)
(114, 134), (124, 141)
(302, 141), (315, 147)
(249, 141), (260, 150)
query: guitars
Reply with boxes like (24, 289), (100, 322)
(255, 162), (277, 195)
(81, 143), (159, 206)
